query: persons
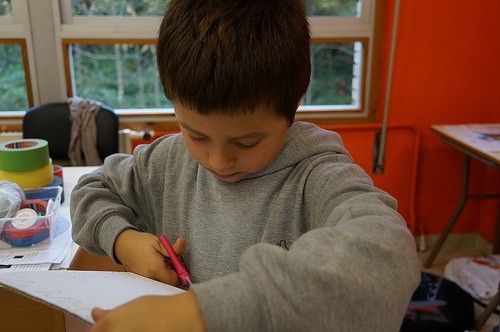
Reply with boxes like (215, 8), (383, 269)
(70, 0), (421, 332)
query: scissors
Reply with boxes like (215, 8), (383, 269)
(158, 234), (193, 287)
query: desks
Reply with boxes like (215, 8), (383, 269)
(423, 122), (500, 332)
(0, 167), (156, 332)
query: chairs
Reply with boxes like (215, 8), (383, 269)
(22, 99), (120, 167)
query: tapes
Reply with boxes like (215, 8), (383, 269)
(0, 139), (65, 248)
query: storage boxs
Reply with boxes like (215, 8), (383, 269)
(0, 186), (63, 251)
